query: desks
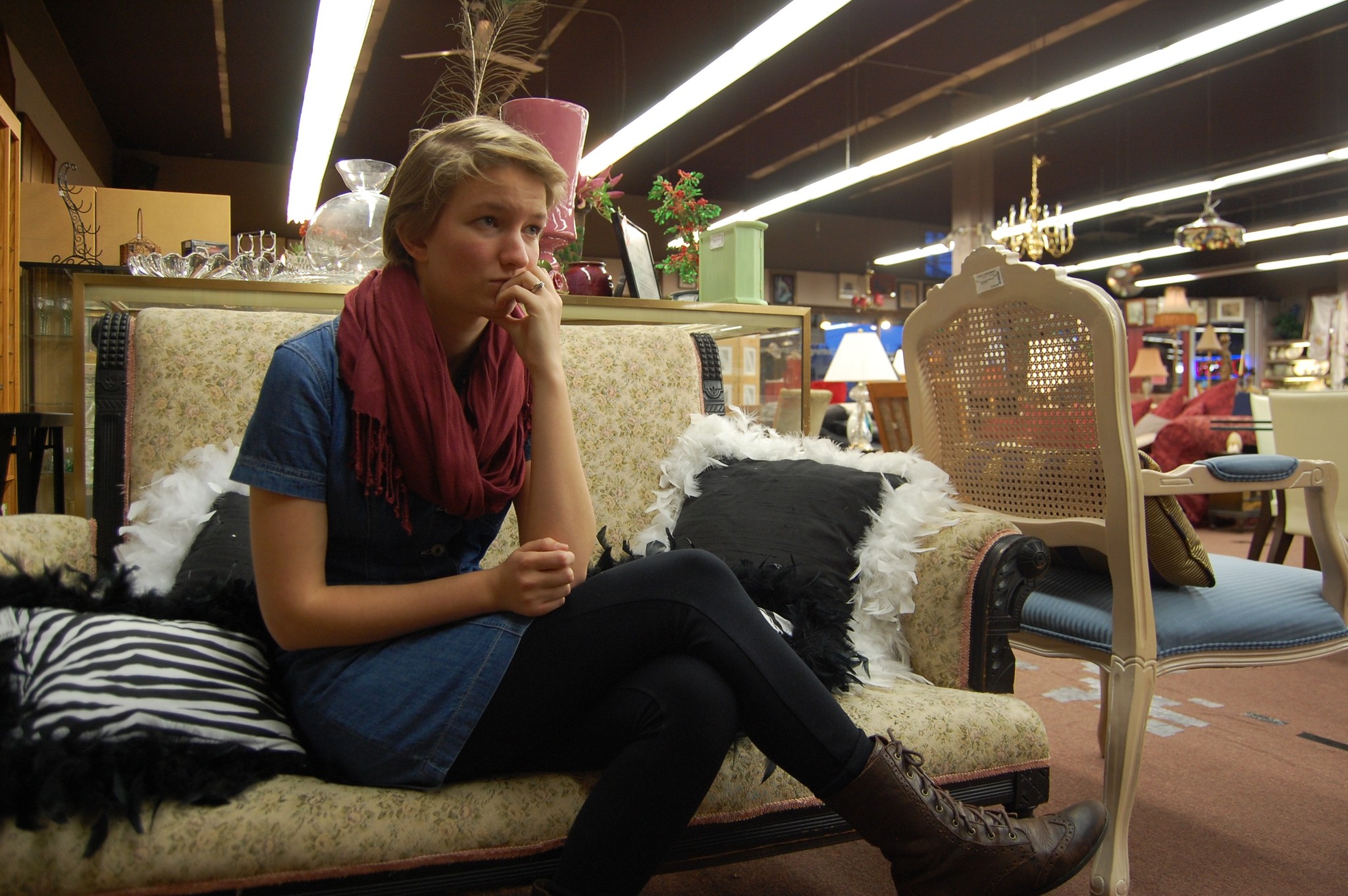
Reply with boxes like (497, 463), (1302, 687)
(69, 269), (814, 523)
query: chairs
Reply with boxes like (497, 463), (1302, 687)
(809, 380), (847, 404)
(864, 379), (969, 453)
(1266, 387), (1348, 565)
(771, 387), (832, 439)
(897, 240), (1348, 896)
(1246, 392), (1316, 570)
(764, 380), (785, 403)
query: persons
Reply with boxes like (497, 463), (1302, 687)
(228, 115), (1111, 896)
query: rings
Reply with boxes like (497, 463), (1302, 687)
(529, 281), (545, 293)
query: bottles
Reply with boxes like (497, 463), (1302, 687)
(305, 158), (391, 274)
(408, 129), (431, 151)
(564, 260), (612, 297)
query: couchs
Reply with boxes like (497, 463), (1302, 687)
(981, 376), (1258, 531)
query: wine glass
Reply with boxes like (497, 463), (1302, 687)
(500, 98), (589, 293)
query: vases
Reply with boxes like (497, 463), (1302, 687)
(564, 260), (614, 297)
(553, 197), (593, 274)
(499, 97), (590, 272)
(669, 289), (700, 302)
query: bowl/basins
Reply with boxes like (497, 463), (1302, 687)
(127, 253), (285, 283)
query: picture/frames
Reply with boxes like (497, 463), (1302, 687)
(1123, 298), (1146, 328)
(611, 211), (664, 299)
(1215, 296), (1247, 322)
(922, 283), (936, 302)
(835, 271), (860, 302)
(897, 281), (919, 310)
(1190, 298), (1209, 324)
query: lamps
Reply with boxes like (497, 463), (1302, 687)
(823, 331), (900, 451)
(1130, 346), (1172, 400)
(282, 0), (1348, 291)
(1194, 322), (1223, 391)
(1151, 286), (1198, 394)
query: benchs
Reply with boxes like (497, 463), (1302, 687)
(0, 302), (1055, 896)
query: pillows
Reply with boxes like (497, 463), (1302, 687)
(629, 402), (963, 695)
(111, 438), (269, 642)
(1071, 401), (1099, 448)
(1182, 377), (1239, 416)
(1175, 399), (1205, 419)
(1150, 384), (1186, 421)
(952, 440), (1218, 589)
(1017, 400), (1075, 450)
(1131, 396), (1154, 427)
(0, 548), (313, 863)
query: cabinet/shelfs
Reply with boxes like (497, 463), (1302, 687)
(15, 260), (132, 516)
(1264, 338), (1321, 384)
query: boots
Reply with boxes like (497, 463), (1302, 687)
(826, 728), (1109, 896)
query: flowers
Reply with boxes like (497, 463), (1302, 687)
(648, 168), (722, 290)
(537, 258), (570, 295)
(576, 163), (624, 223)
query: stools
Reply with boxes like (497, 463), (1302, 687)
(0, 410), (76, 516)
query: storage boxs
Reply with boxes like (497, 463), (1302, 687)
(180, 240), (230, 269)
